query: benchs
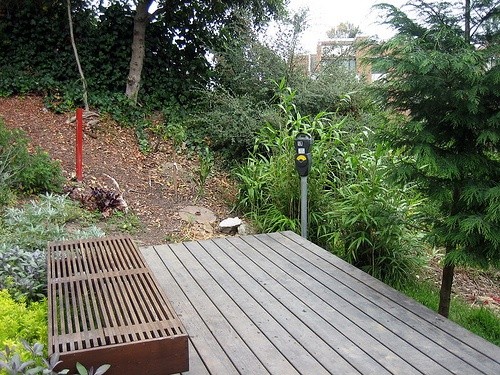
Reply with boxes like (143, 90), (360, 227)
(46, 234), (189, 375)
